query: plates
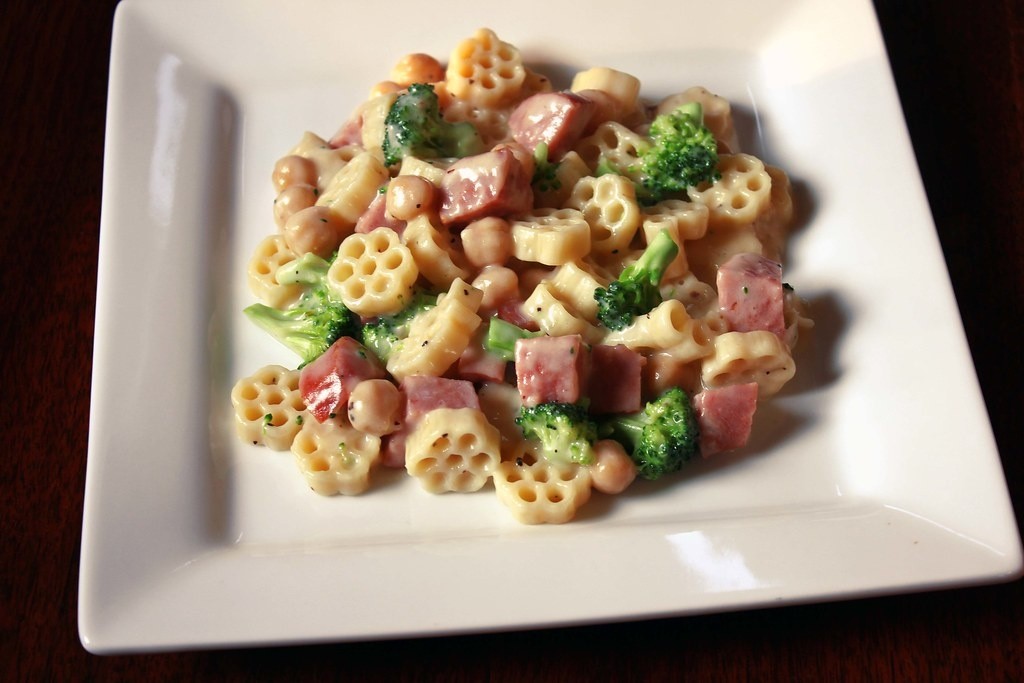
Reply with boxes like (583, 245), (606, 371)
(75, 0), (1023, 657)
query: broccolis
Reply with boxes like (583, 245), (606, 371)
(242, 84), (724, 483)
